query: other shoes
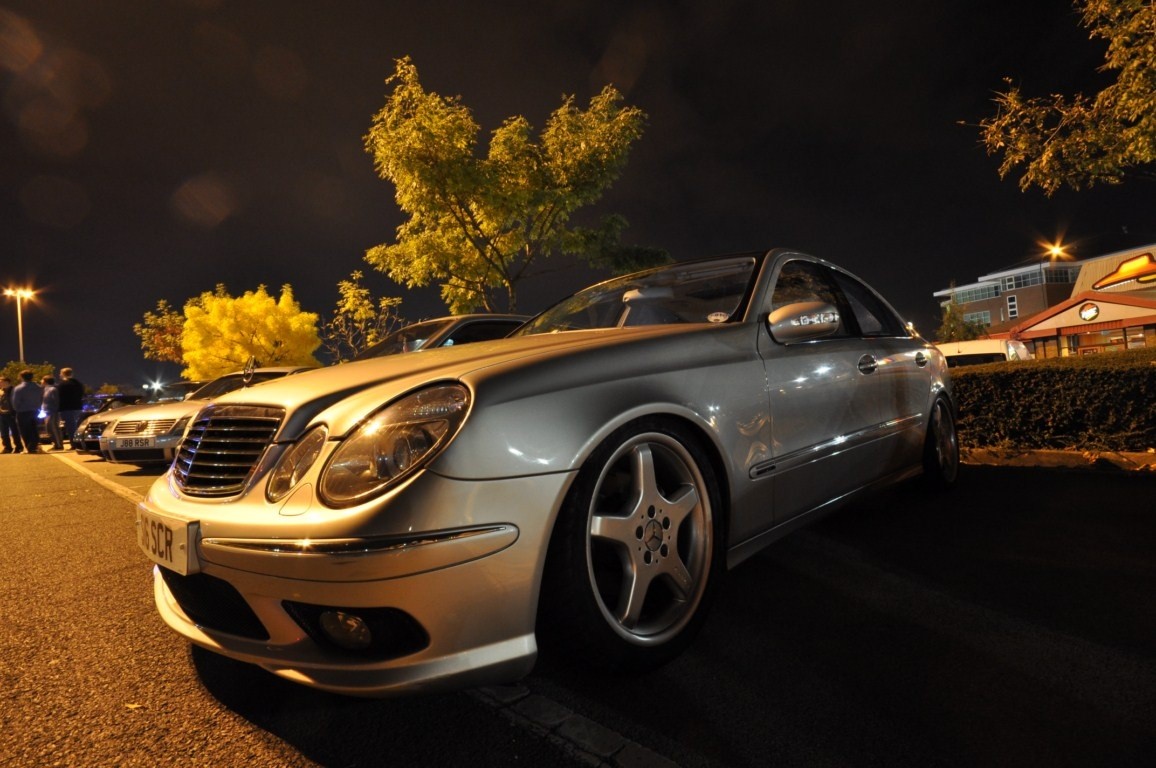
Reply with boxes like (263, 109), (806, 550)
(29, 449), (38, 454)
(0, 447), (12, 453)
(49, 446), (64, 450)
(14, 445), (23, 453)
(68, 446), (76, 450)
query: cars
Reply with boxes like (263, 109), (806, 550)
(45, 394), (145, 441)
(72, 380), (212, 460)
(134, 249), (961, 699)
(98, 363), (318, 473)
(933, 338), (1031, 370)
(347, 314), (535, 362)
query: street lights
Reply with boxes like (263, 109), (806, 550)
(3, 288), (32, 363)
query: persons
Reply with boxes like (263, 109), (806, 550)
(0, 376), (23, 454)
(58, 367), (85, 450)
(40, 374), (64, 451)
(12, 370), (44, 453)
